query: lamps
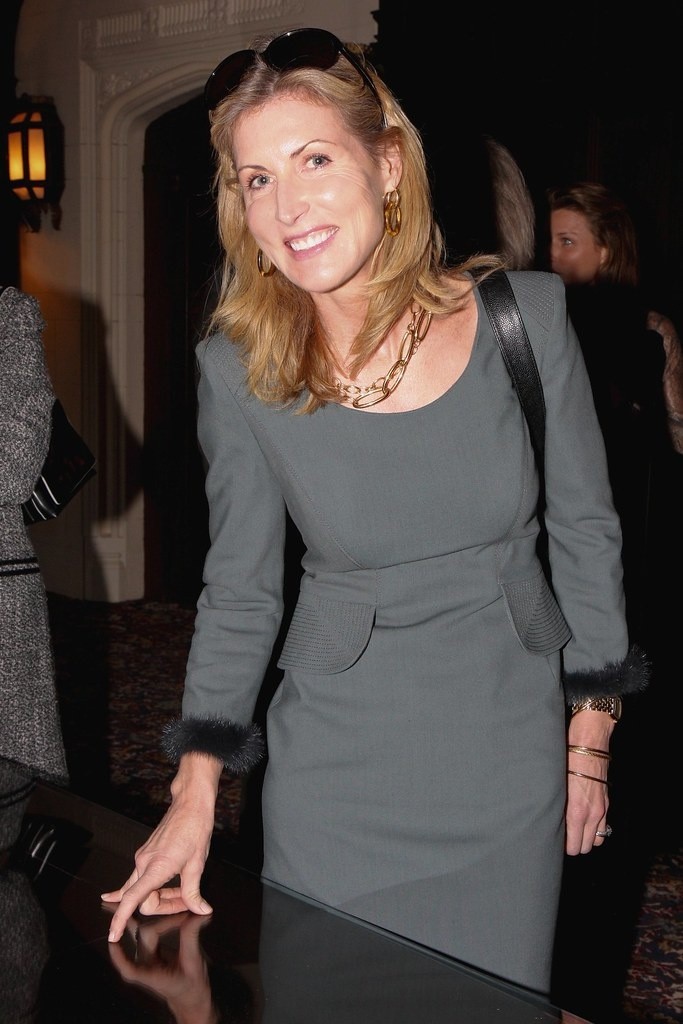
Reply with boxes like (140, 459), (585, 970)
(1, 92), (66, 236)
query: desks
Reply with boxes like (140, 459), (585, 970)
(0, 741), (615, 1024)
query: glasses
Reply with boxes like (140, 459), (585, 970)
(202, 27), (386, 127)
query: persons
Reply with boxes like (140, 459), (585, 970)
(2, 251), (74, 839)
(103, 23), (644, 1018)
(428, 140), (537, 272)
(529, 184), (683, 899)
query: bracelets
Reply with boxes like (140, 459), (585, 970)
(562, 741), (616, 790)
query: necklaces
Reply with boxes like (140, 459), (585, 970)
(283, 262), (437, 412)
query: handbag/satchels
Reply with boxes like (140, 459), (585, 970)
(21, 395), (97, 526)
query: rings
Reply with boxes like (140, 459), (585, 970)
(595, 825), (613, 838)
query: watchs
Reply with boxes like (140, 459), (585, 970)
(569, 693), (624, 723)
